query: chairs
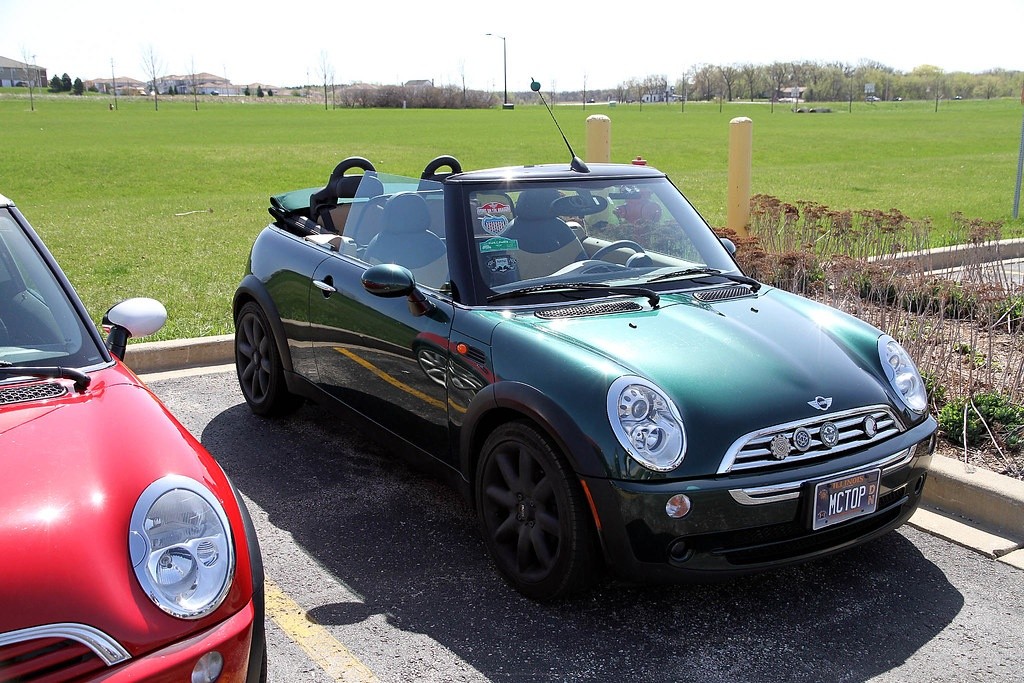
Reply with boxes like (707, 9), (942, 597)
(318, 155), (388, 248)
(492, 188), (593, 285)
(360, 190), (449, 292)
(420, 155), (488, 240)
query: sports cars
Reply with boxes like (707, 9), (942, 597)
(236, 159), (939, 604)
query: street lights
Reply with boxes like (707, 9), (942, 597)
(486, 33), (508, 109)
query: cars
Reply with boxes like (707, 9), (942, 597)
(2, 192), (266, 683)
